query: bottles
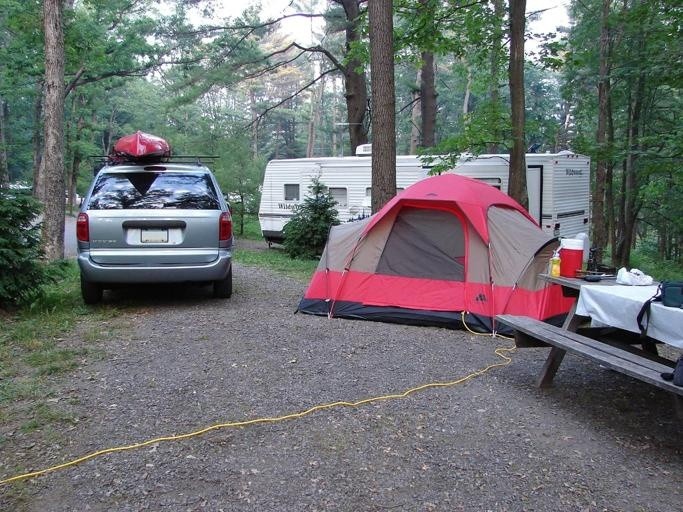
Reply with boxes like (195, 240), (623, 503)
(551, 253), (561, 277)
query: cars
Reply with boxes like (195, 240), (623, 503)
(64, 189), (86, 210)
(1, 179), (34, 194)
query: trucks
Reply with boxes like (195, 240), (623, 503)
(224, 178), (263, 215)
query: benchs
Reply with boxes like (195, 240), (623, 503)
(493, 314), (683, 399)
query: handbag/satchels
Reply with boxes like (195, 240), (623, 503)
(661, 355), (683, 386)
(659, 280), (683, 309)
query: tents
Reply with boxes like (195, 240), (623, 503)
(293, 172), (578, 337)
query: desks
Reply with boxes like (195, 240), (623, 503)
(533, 273), (683, 389)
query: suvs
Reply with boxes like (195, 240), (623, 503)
(73, 144), (233, 305)
